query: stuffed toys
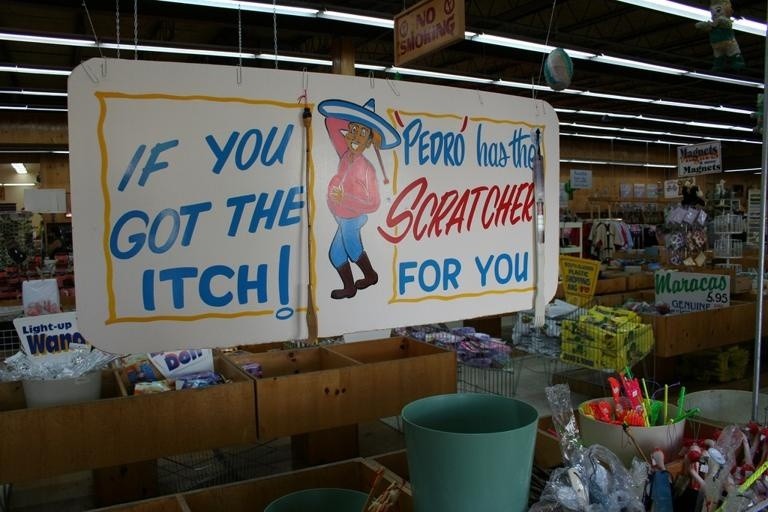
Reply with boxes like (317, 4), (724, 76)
(695, 0), (746, 73)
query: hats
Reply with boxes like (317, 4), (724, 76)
(319, 98), (401, 149)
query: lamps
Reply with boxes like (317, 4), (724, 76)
(0, 1), (768, 174)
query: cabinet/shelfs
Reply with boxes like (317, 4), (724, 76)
(0, 272), (456, 512)
(539, 251), (767, 465)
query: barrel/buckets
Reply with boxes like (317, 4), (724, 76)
(678, 389), (768, 428)
(23, 374), (101, 410)
(577, 397), (686, 470)
(260, 487), (387, 512)
(402, 394), (539, 511)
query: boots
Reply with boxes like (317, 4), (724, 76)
(331, 262), (356, 299)
(355, 252), (379, 290)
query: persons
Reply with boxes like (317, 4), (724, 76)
(318, 99), (401, 299)
